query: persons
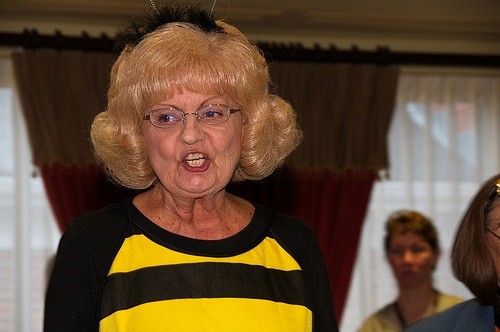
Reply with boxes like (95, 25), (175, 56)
(41, 20), (341, 331)
(355, 209), (465, 332)
(398, 174), (500, 331)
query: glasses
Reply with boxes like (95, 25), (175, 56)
(140, 105), (243, 128)
